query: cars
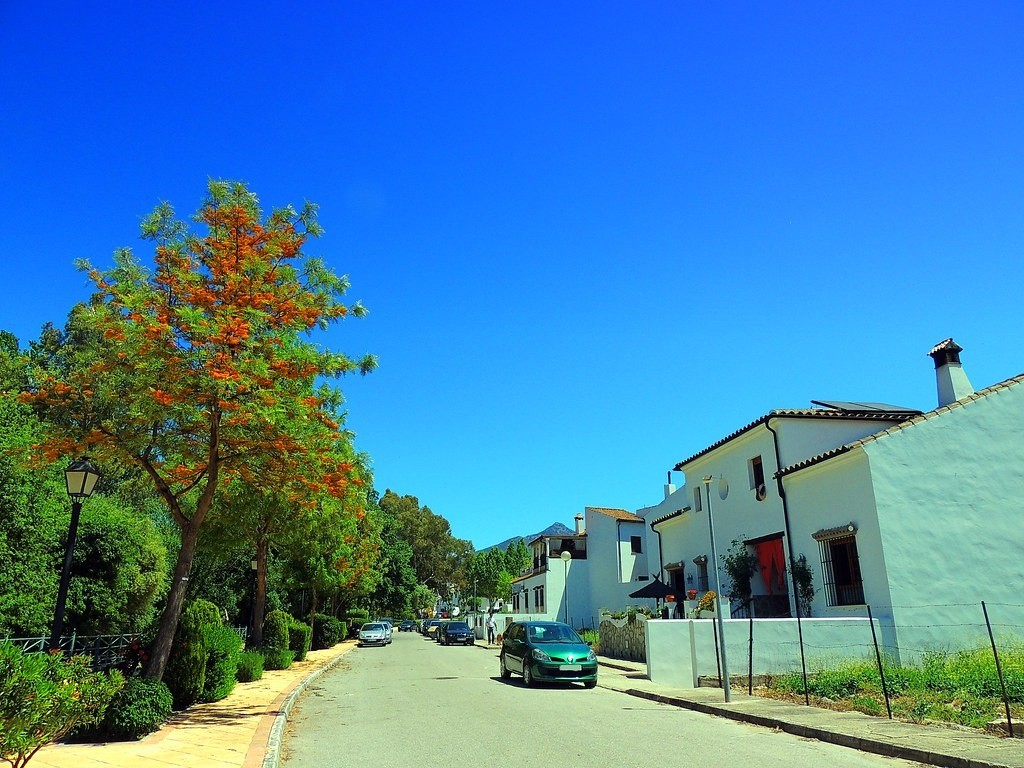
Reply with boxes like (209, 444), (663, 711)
(398, 620), (418, 632)
(499, 620), (598, 689)
(438, 612), (450, 618)
(357, 617), (394, 646)
(420, 619), (475, 646)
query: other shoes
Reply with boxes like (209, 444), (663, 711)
(488, 642), (490, 645)
(492, 642), (495, 644)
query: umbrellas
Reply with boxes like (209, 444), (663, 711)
(629, 579), (677, 612)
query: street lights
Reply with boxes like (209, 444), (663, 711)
(703, 472), (731, 702)
(49, 455), (101, 653)
(561, 551), (571, 624)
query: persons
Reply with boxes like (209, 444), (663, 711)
(486, 613), (498, 645)
